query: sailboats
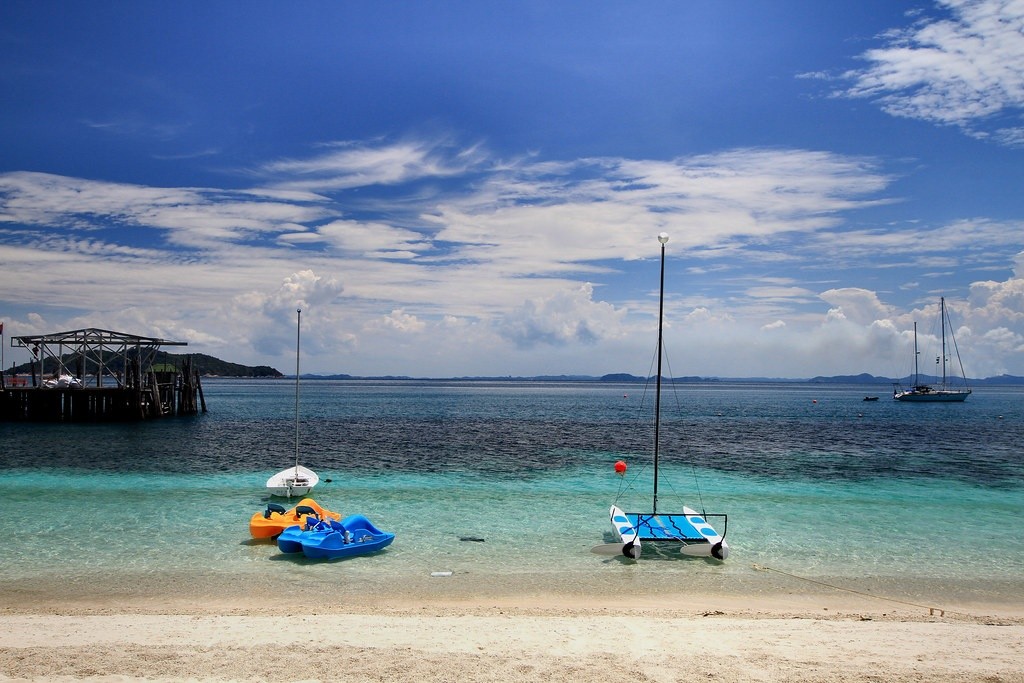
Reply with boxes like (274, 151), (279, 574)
(267, 308), (320, 498)
(610, 232), (730, 560)
(892, 296), (972, 402)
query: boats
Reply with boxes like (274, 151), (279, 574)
(42, 374), (83, 389)
(276, 514), (395, 561)
(250, 498), (341, 541)
(863, 396), (879, 400)
